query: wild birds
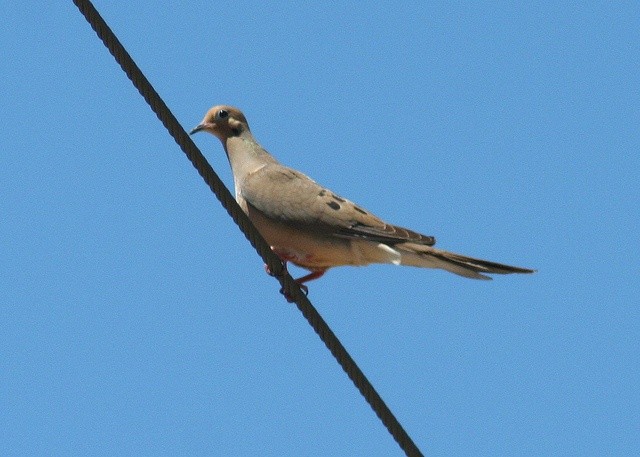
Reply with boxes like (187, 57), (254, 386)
(189, 104), (538, 304)
